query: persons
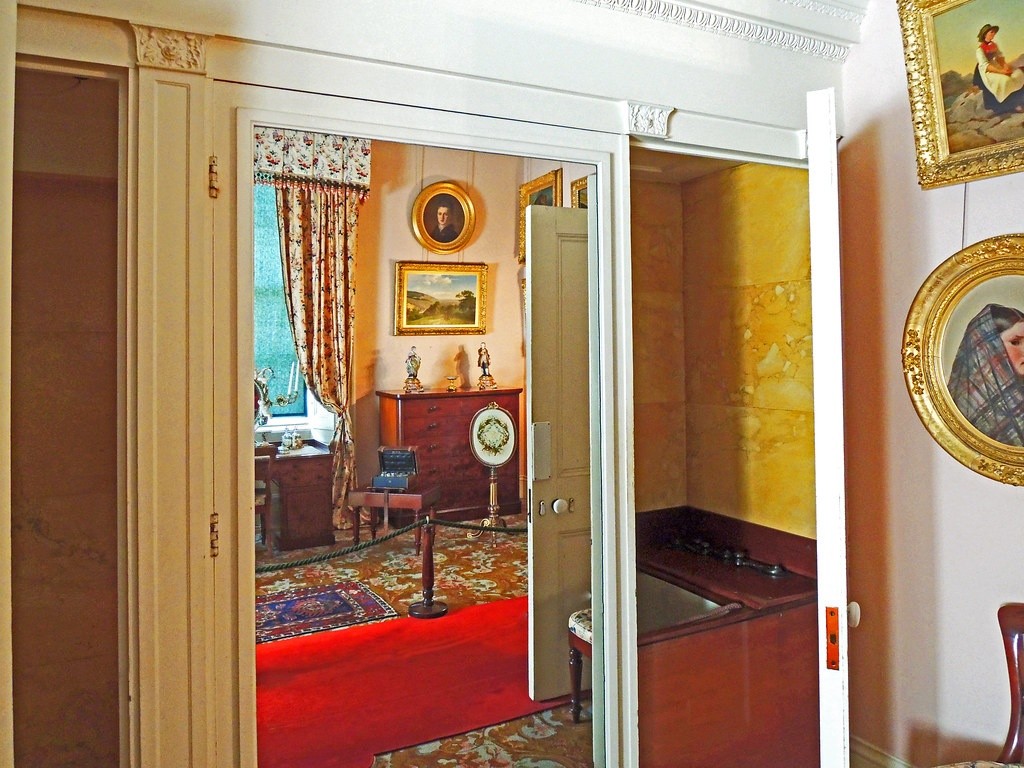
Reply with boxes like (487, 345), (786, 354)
(478, 342), (492, 375)
(405, 346), (421, 378)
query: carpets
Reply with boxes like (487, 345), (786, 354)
(256, 595), (596, 768)
(255, 580), (400, 644)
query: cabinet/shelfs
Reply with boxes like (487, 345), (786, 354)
(373, 387), (523, 529)
(254, 439), (335, 551)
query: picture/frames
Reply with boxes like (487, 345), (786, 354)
(411, 182), (476, 256)
(517, 168), (564, 263)
(394, 260), (488, 336)
(901, 231), (1024, 489)
(571, 175), (589, 210)
(895, 0), (1024, 192)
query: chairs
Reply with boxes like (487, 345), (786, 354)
(255, 444), (279, 562)
(929, 602), (1024, 768)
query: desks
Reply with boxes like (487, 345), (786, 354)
(347, 480), (442, 556)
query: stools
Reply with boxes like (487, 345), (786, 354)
(565, 607), (592, 724)
